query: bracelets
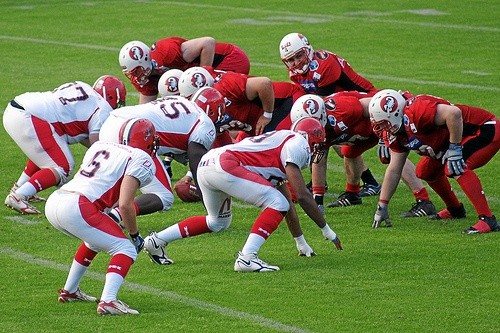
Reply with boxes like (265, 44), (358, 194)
(263, 111), (273, 118)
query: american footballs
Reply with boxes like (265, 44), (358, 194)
(175, 182), (203, 202)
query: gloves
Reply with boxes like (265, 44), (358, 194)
(408, 136), (442, 159)
(162, 160), (173, 179)
(442, 144), (467, 175)
(378, 139), (392, 164)
(372, 202), (391, 229)
(129, 232), (145, 254)
(294, 223), (343, 258)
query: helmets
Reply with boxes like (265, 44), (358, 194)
(119, 41), (152, 87)
(92, 75), (127, 110)
(279, 33), (315, 75)
(119, 118), (161, 158)
(291, 94), (327, 164)
(158, 67), (226, 124)
(369, 89), (407, 141)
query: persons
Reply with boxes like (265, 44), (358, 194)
(119, 37), (250, 105)
(45, 118), (160, 315)
(290, 90), (436, 218)
(279, 32), (382, 202)
(368, 89), (500, 234)
(143, 117), (342, 273)
(2, 75), (127, 215)
(99, 88), (226, 229)
(158, 66), (306, 184)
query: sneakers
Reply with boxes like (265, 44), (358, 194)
(143, 231), (174, 265)
(5, 182), (46, 215)
(327, 191), (362, 208)
(400, 199), (436, 217)
(96, 299), (139, 315)
(305, 179), (328, 193)
(462, 215), (500, 234)
(58, 287), (97, 303)
(234, 251), (281, 272)
(358, 183), (382, 197)
(429, 203), (466, 220)
